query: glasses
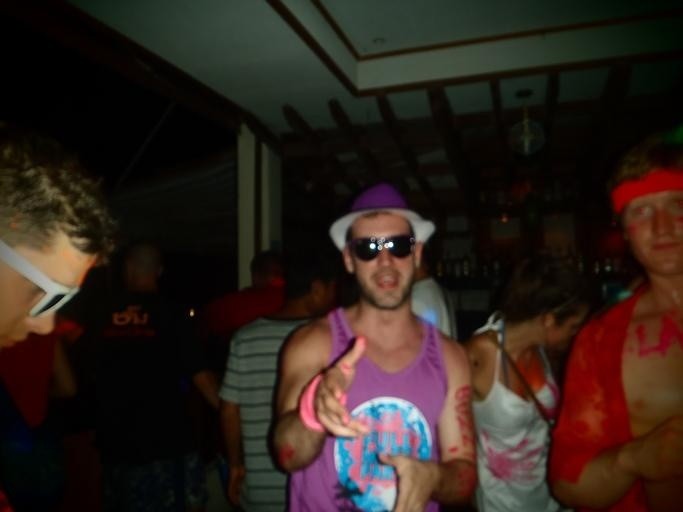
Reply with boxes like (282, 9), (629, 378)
(348, 233), (415, 262)
(0, 241), (81, 320)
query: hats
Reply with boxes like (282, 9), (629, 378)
(327, 182), (437, 253)
(609, 139), (683, 215)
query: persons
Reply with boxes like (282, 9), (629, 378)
(1, 142), (682, 512)
